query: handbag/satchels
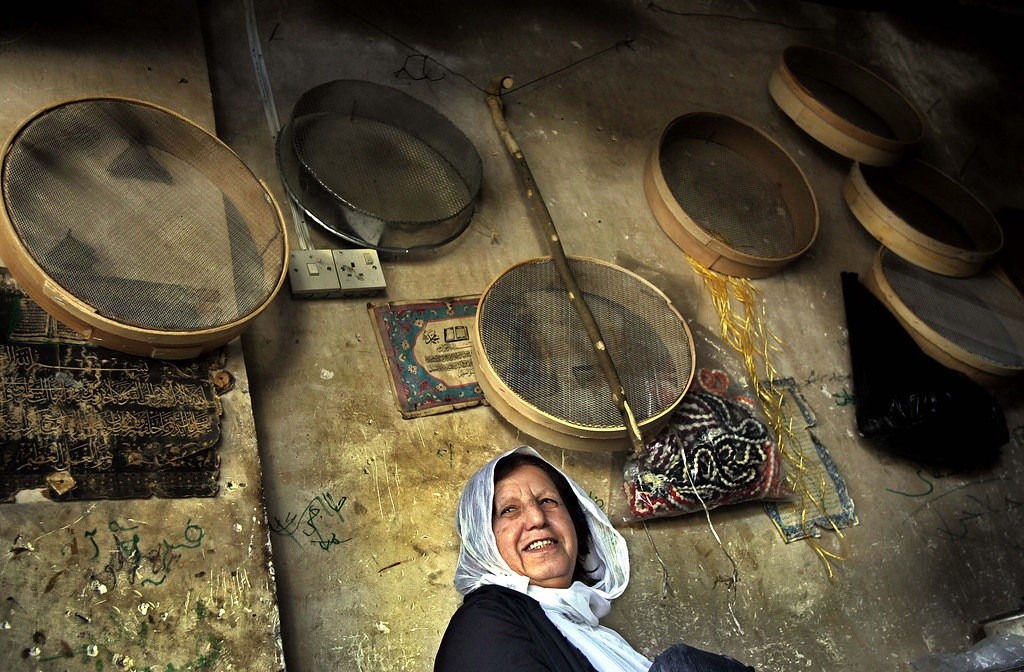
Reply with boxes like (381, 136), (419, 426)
(840, 269), (1011, 467)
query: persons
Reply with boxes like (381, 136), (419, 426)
(431, 444), (760, 672)
